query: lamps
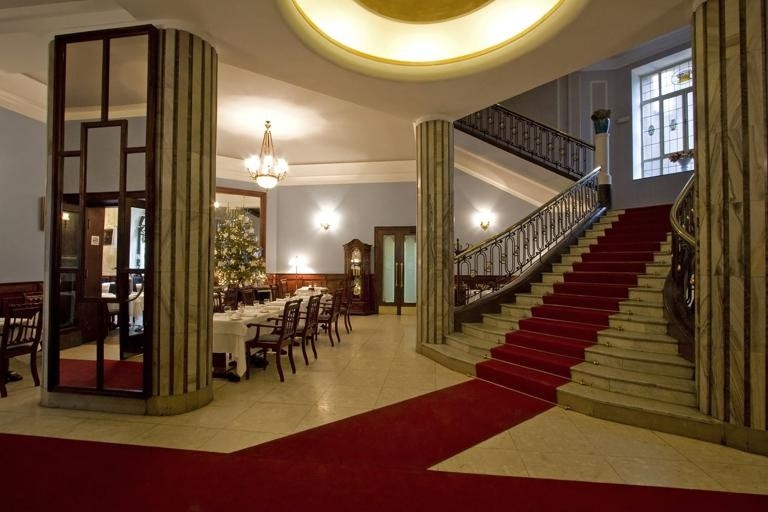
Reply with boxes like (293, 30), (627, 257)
(480, 219), (491, 231)
(275, 1), (588, 83)
(243, 120), (289, 189)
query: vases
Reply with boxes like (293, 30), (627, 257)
(594, 117), (610, 134)
(678, 158), (691, 172)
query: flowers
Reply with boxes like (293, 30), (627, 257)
(591, 109), (612, 122)
(214, 207), (269, 288)
(668, 149), (697, 163)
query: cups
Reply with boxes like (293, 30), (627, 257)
(264, 299), (269, 304)
(285, 293), (290, 298)
(253, 300), (259, 306)
(224, 305), (231, 313)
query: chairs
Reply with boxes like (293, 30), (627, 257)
(0, 303), (43, 398)
(212, 279), (357, 383)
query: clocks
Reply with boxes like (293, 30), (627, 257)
(342, 239), (373, 316)
